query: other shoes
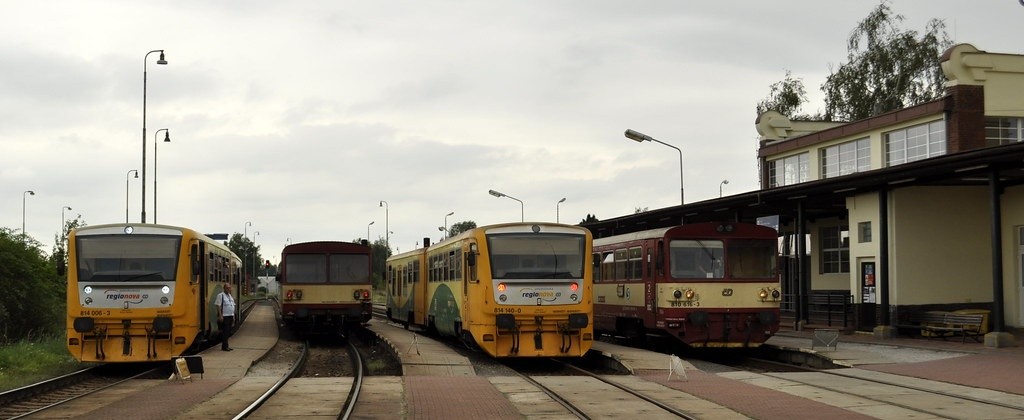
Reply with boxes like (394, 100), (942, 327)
(223, 347), (233, 351)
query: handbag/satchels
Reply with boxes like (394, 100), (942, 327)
(217, 319), (223, 331)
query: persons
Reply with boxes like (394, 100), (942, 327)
(215, 283), (236, 352)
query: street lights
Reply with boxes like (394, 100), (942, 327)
(368, 221), (374, 240)
(379, 201), (389, 258)
(439, 226), (450, 234)
(623, 128), (687, 206)
(244, 221), (251, 296)
(126, 169), (139, 224)
(489, 189), (524, 222)
(252, 231), (260, 278)
(557, 198), (567, 223)
(140, 47), (171, 225)
(22, 190), (35, 242)
(444, 212), (455, 240)
(719, 180), (730, 197)
(287, 237), (292, 245)
(61, 206), (72, 238)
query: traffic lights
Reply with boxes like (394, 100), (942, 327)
(266, 260), (269, 269)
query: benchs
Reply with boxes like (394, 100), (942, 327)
(921, 309), (991, 343)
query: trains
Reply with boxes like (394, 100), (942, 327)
(385, 224), (595, 360)
(593, 224), (782, 352)
(66, 222), (245, 365)
(274, 240), (373, 333)
